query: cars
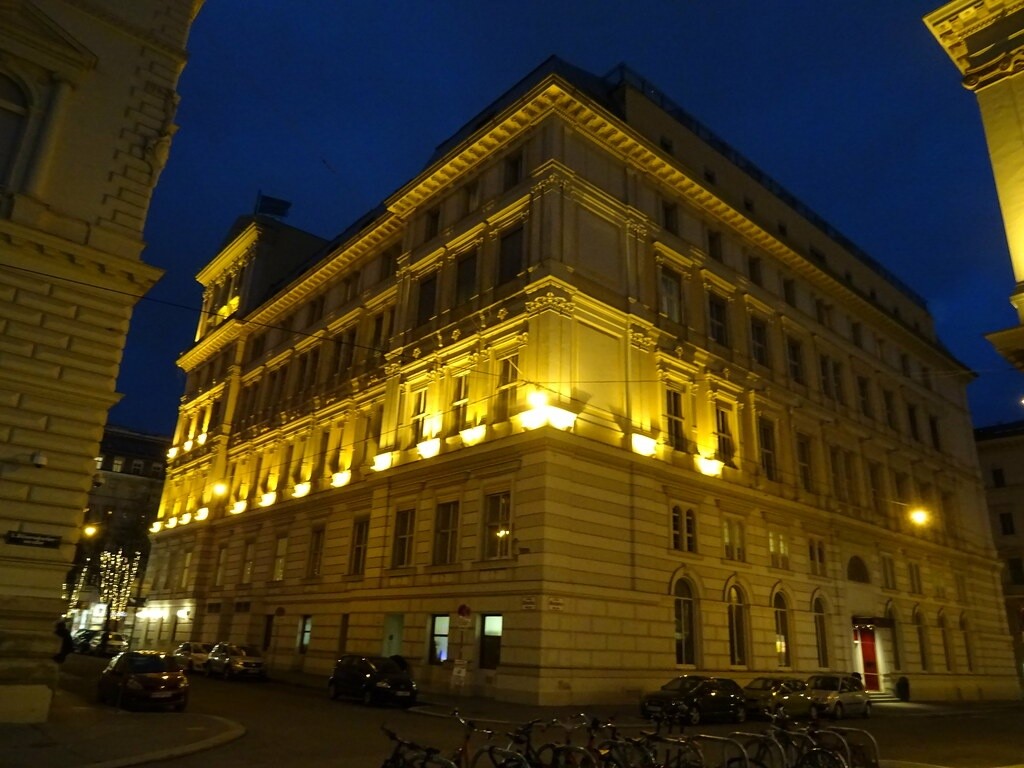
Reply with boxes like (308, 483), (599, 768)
(89, 630), (128, 649)
(740, 676), (818, 723)
(806, 673), (874, 717)
(70, 630), (98, 654)
(173, 642), (215, 673)
(206, 642), (266, 681)
(97, 648), (189, 712)
(639, 676), (750, 727)
(326, 655), (418, 709)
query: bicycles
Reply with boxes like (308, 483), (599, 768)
(376, 708), (876, 768)
